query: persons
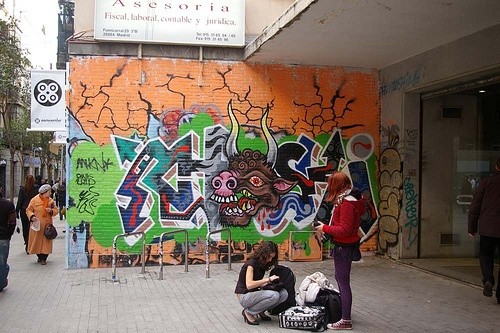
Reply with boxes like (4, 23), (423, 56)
(234, 241), (288, 325)
(16, 174), (39, 255)
(0, 186), (16, 293)
(468, 158), (500, 304)
(40, 176), (66, 220)
(26, 184), (59, 265)
(311, 172), (365, 330)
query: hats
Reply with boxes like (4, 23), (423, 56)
(38, 184), (51, 193)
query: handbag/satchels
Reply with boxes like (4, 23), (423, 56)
(352, 243), (361, 261)
(262, 279), (284, 290)
(44, 223), (58, 240)
(305, 288), (342, 323)
(17, 227), (20, 233)
(60, 207), (66, 215)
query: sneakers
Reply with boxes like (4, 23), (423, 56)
(326, 318), (352, 329)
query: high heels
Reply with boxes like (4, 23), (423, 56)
(256, 312), (271, 321)
(242, 308), (259, 325)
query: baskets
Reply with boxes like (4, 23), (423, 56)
(278, 304), (325, 329)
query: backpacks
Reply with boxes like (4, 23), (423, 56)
(267, 265), (296, 316)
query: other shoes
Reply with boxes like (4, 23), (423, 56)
(496, 298), (500, 304)
(41, 260), (46, 265)
(483, 281), (492, 297)
(0, 279), (8, 292)
(38, 259), (41, 262)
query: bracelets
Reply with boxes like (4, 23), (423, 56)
(268, 277), (271, 282)
